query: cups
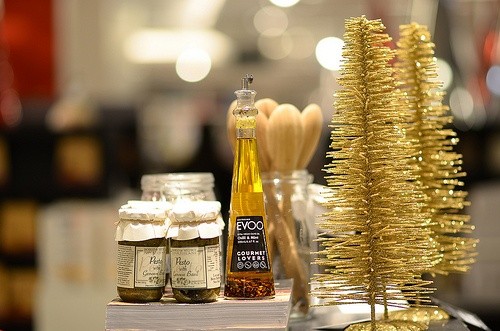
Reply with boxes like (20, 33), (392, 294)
(140, 174), (215, 201)
(260, 170), (319, 289)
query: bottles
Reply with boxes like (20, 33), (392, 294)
(225, 78), (276, 299)
